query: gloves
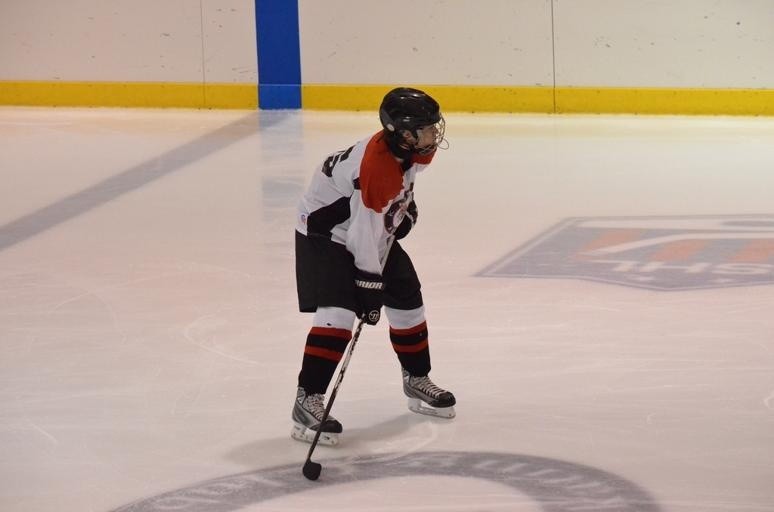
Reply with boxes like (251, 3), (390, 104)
(385, 197), (418, 238)
(351, 270), (388, 325)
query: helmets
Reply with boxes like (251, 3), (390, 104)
(378, 87), (446, 157)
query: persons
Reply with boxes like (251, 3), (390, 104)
(291, 88), (457, 433)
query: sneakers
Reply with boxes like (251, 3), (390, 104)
(401, 368), (456, 408)
(292, 388), (342, 433)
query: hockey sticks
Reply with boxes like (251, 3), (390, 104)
(302, 239), (394, 482)
